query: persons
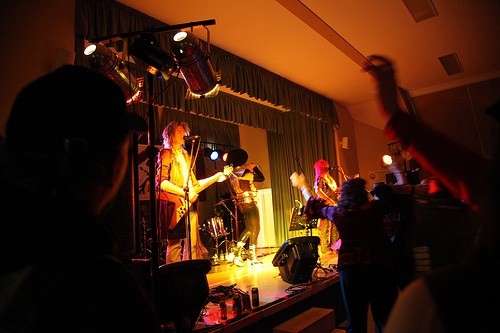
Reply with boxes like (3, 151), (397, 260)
(313, 159), (340, 256)
(225, 148), (266, 267)
(382, 137), (500, 333)
(360, 52), (500, 214)
(290, 175), (415, 333)
(0, 63), (211, 333)
(155, 121), (226, 267)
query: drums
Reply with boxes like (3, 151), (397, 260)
(206, 217), (224, 238)
(236, 190), (259, 204)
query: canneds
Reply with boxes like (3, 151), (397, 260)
(251, 287), (260, 307)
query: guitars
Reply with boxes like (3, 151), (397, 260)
(154, 164), (234, 230)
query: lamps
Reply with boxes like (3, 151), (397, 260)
(383, 143), (400, 166)
(127, 30), (176, 81)
(84, 39), (138, 104)
(203, 143), (219, 161)
(172, 22), (222, 100)
(215, 145), (228, 162)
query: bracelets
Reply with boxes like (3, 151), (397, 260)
(301, 185), (308, 191)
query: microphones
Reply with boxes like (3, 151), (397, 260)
(328, 167), (340, 170)
(183, 135), (199, 141)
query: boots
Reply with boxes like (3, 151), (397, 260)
(227, 242), (245, 266)
(247, 245), (263, 264)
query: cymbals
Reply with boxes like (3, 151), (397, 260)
(213, 198), (232, 205)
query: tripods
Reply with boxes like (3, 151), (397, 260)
(218, 203), (247, 255)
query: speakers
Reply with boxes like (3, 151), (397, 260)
(153, 259), (212, 327)
(272, 236), (321, 285)
